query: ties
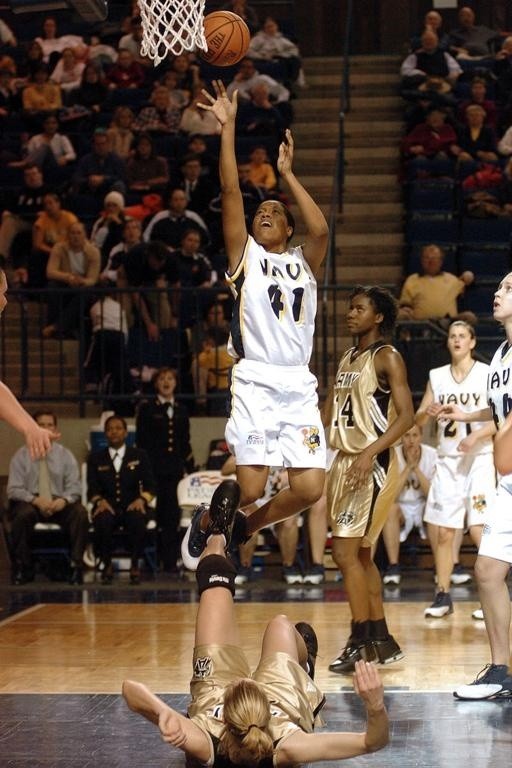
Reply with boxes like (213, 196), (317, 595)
(38, 456), (55, 515)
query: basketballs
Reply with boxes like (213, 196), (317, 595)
(200, 12), (251, 66)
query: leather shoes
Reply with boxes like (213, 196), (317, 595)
(5, 564), (142, 585)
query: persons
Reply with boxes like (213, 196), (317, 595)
(220, 454), (344, 585)
(87, 417), (157, 587)
(321, 285), (415, 674)
(135, 370), (196, 572)
(398, 6), (512, 217)
(7, 408), (90, 587)
(182, 79), (330, 571)
(0, 272), (61, 461)
(2, 0), (308, 411)
(122, 480), (390, 768)
(380, 244), (512, 702)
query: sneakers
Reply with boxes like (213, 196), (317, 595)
(281, 560), (325, 586)
(433, 561), (472, 585)
(453, 661), (512, 701)
(181, 502), (210, 571)
(235, 564), (251, 585)
(328, 631), (405, 673)
(295, 622), (319, 681)
(424, 592), (454, 619)
(381, 561), (402, 585)
(204, 477), (242, 543)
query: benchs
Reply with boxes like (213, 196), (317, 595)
(0, 34), (265, 410)
(392, 28), (512, 411)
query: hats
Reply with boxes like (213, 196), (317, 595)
(102, 190), (125, 210)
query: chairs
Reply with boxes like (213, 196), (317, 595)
(81, 464), (155, 586)
(4, 473), (87, 574)
